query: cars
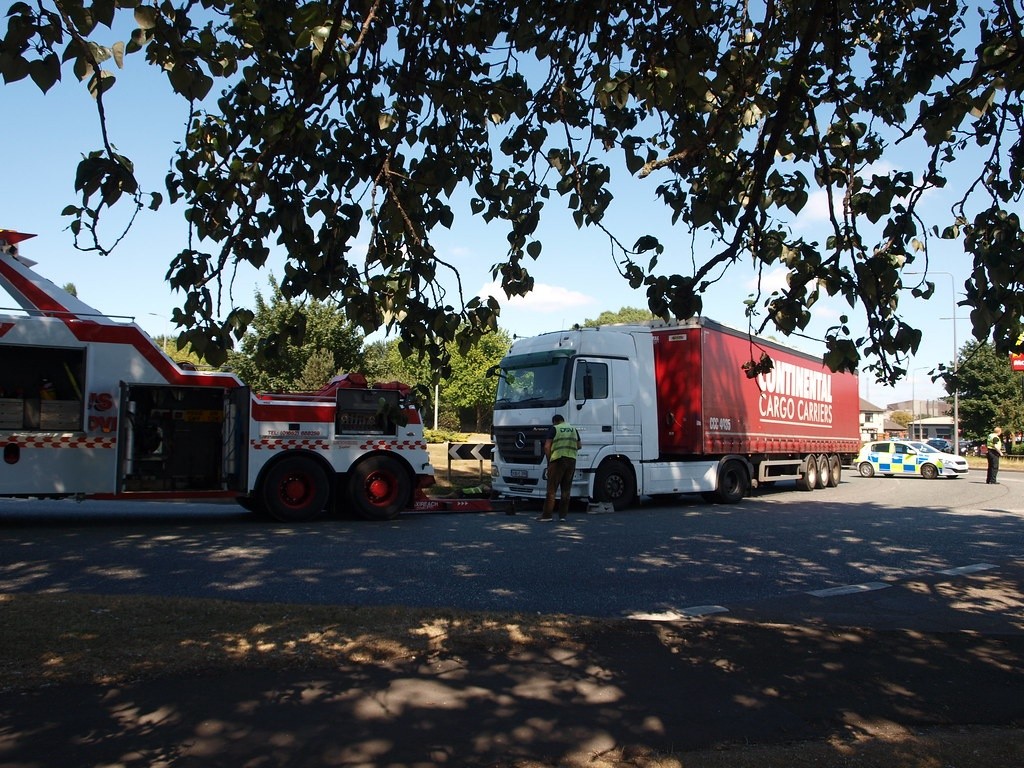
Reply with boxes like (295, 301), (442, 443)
(953, 441), (975, 456)
(853, 438), (969, 479)
(926, 438), (951, 452)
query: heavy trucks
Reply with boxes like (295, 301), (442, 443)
(486, 316), (861, 512)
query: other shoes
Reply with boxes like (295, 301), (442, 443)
(986, 481), (1001, 484)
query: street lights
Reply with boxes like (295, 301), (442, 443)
(912, 366), (929, 440)
(904, 271), (959, 455)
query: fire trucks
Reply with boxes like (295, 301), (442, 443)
(0, 226), (435, 522)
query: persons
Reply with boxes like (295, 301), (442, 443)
(536, 415), (582, 522)
(986, 427), (1004, 484)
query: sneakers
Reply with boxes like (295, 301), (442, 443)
(559, 516), (566, 521)
(536, 516), (553, 522)
